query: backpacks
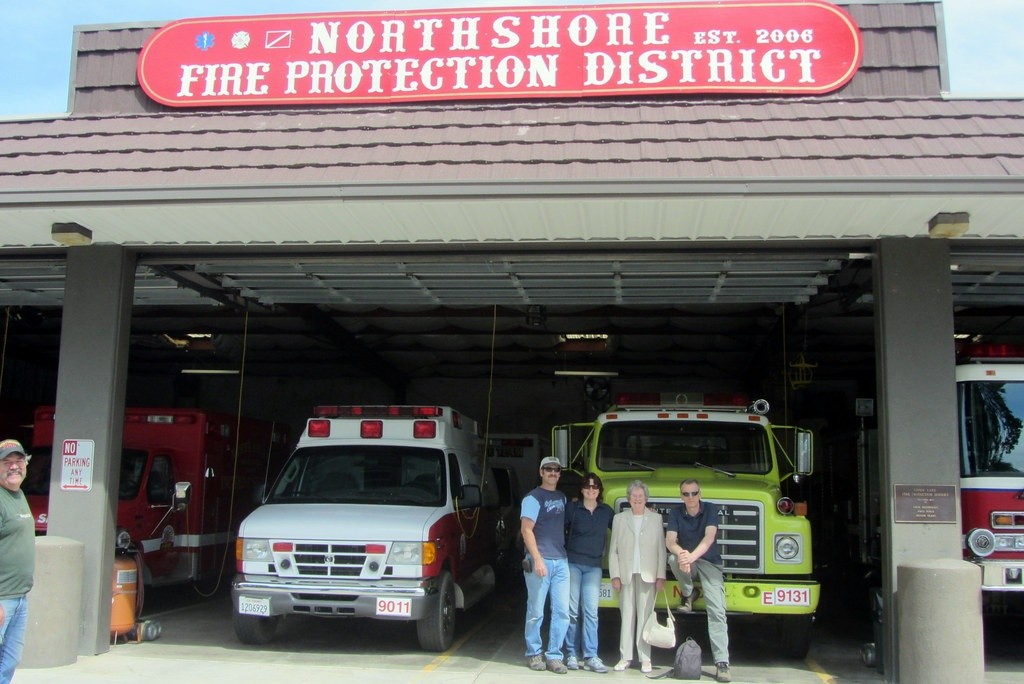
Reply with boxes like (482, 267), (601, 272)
(645, 636), (701, 680)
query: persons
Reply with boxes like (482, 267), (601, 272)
(666, 477), (731, 683)
(608, 480), (667, 673)
(0, 439), (36, 684)
(520, 457), (570, 674)
(564, 473), (616, 673)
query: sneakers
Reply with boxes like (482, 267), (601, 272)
(527, 654), (546, 671)
(677, 588), (700, 612)
(584, 656), (610, 673)
(545, 659), (568, 674)
(567, 656), (580, 669)
(715, 662), (730, 682)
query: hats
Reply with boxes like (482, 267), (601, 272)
(540, 457), (562, 468)
(0, 439), (26, 461)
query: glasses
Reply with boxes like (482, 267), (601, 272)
(680, 489), (699, 496)
(581, 483), (599, 490)
(542, 466), (561, 473)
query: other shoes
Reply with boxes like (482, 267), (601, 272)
(614, 660), (631, 670)
(641, 661), (652, 673)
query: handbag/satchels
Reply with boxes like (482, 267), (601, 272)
(643, 611), (676, 648)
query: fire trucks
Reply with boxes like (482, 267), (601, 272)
(836, 347), (1024, 670)
(230, 403), (551, 654)
(16, 400), (295, 608)
(549, 391), (823, 659)
